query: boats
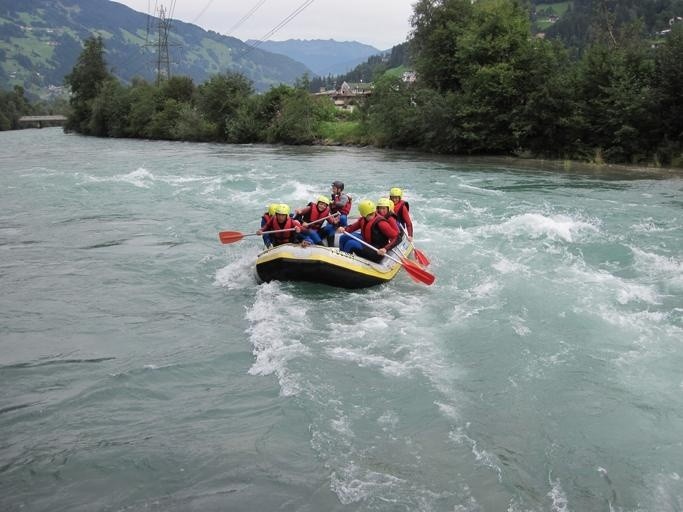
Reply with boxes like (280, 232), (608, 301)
(253, 231), (415, 287)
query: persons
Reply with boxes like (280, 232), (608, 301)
(383, 186), (413, 243)
(375, 197), (402, 248)
(293, 196), (336, 247)
(324, 180), (350, 229)
(339, 199), (398, 263)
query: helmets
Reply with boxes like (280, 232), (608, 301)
(331, 181), (344, 190)
(358, 200), (377, 217)
(376, 198), (394, 214)
(318, 196), (330, 206)
(390, 187), (402, 198)
(268, 202), (289, 217)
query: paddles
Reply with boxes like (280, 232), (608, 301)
(343, 223), (435, 287)
(218, 226), (295, 244)
